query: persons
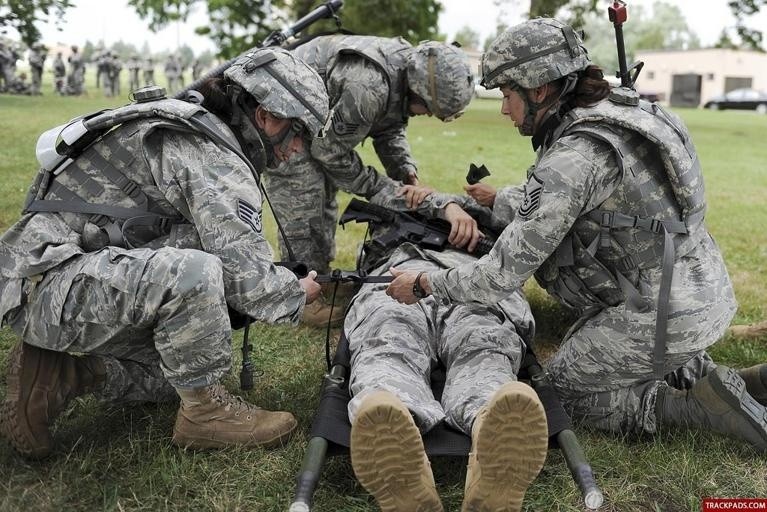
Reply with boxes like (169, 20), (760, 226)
(327, 186), (549, 512)
(384, 18), (766, 453)
(464, 178), (531, 231)
(0, 44), (323, 458)
(0, 43), (203, 96)
(262, 32), (474, 329)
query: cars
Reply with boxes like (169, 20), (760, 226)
(700, 86), (767, 113)
(472, 74), (505, 100)
(605, 77), (664, 105)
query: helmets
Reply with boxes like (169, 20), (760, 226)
(406, 39), (476, 123)
(225, 47), (330, 140)
(478, 17), (590, 90)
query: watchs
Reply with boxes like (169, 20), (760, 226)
(411, 271), (429, 298)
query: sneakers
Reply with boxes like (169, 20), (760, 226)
(321, 267), (358, 298)
(299, 293), (345, 328)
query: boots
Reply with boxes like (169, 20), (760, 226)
(2, 337), (107, 462)
(656, 367), (766, 452)
(170, 382), (298, 451)
(461, 379), (550, 512)
(736, 361), (766, 404)
(348, 391), (443, 512)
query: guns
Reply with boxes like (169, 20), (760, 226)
(171, 0), (344, 98)
(338, 196), (497, 258)
